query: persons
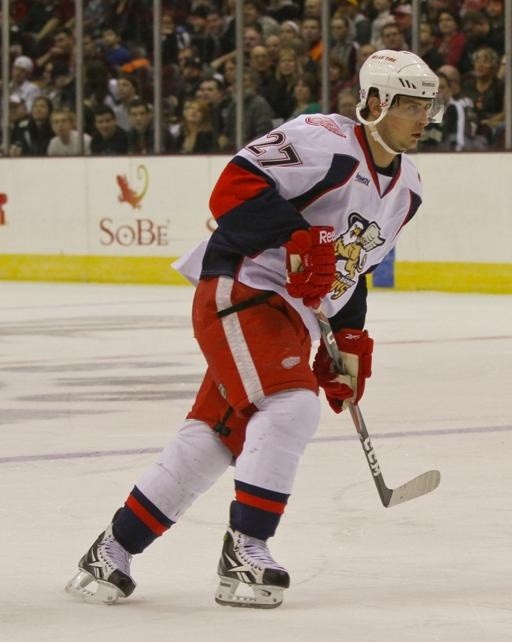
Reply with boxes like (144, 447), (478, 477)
(78, 49), (440, 597)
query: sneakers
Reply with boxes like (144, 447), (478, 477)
(76, 520), (135, 597)
(215, 528), (293, 591)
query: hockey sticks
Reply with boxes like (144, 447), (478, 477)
(287, 253), (440, 509)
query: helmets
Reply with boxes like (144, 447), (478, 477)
(355, 49), (441, 125)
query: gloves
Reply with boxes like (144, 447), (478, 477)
(283, 225), (339, 310)
(313, 326), (374, 414)
